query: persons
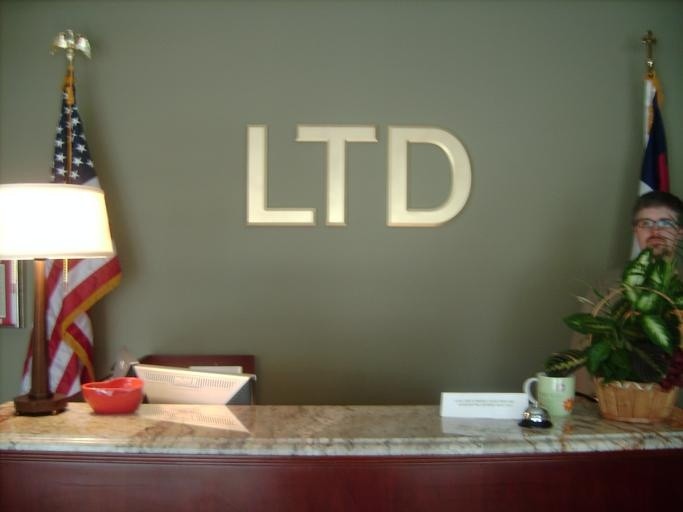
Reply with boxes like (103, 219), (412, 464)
(631, 192), (683, 277)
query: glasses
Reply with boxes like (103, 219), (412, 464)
(633, 217), (672, 227)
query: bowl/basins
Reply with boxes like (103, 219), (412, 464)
(81, 376), (144, 414)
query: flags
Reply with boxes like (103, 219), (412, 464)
(20, 68), (124, 402)
(630, 71), (670, 263)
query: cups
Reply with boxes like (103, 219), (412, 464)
(523, 371), (575, 416)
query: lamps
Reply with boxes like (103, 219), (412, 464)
(0, 183), (116, 416)
